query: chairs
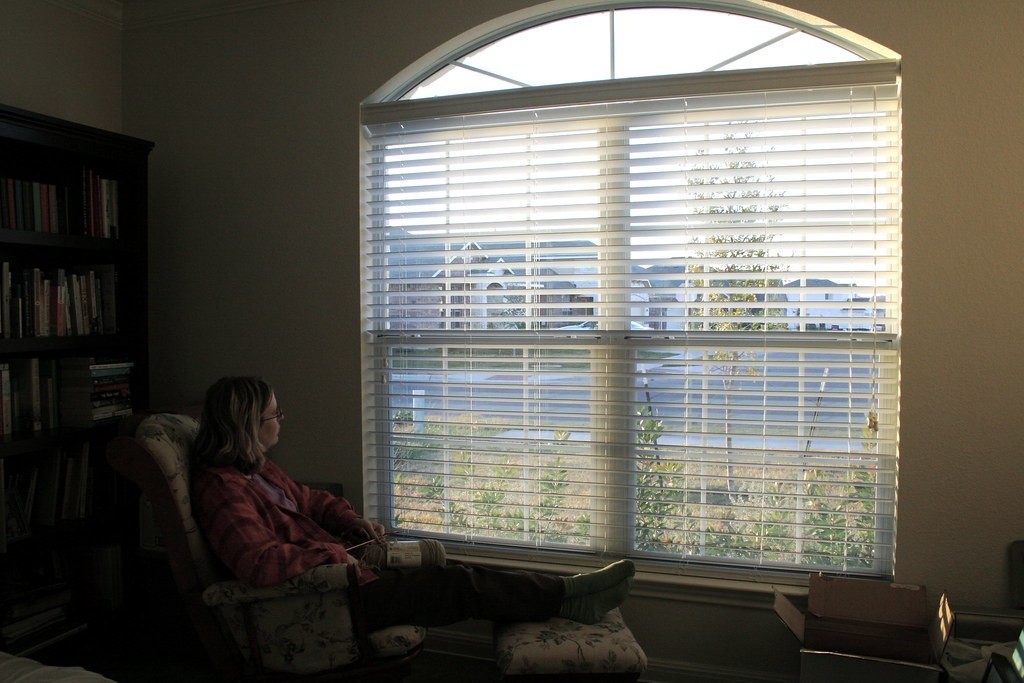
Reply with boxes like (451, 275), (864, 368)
(136, 413), (429, 683)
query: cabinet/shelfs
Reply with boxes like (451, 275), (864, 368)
(0, 105), (156, 669)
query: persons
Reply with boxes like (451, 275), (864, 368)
(190, 376), (635, 632)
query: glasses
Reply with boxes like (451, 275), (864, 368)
(260, 407), (283, 420)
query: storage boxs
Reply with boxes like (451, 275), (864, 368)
(771, 572), (955, 683)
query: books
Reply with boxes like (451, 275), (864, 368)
(1, 357), (134, 435)
(0, 262), (118, 339)
(0, 442), (90, 553)
(0, 164), (119, 238)
(2, 545), (122, 656)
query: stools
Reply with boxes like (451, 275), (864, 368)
(492, 606), (649, 683)
